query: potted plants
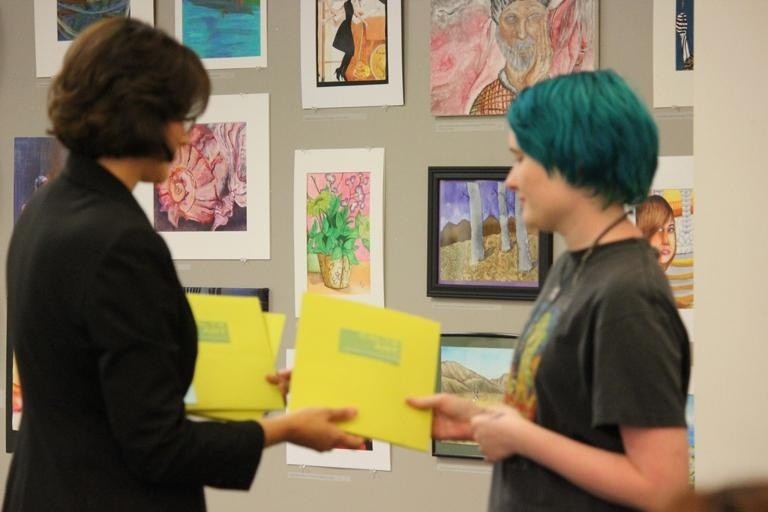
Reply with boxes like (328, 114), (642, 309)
(306, 170), (371, 290)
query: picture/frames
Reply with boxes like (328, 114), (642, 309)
(285, 346), (391, 474)
(174, 1), (269, 70)
(33, 1), (157, 80)
(130, 92), (273, 260)
(300, 0), (405, 108)
(651, 0), (694, 109)
(295, 147), (387, 315)
(427, 166), (548, 300)
(432, 327), (523, 464)
(623, 156), (695, 344)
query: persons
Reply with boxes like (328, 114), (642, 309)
(636, 195), (677, 268)
(405, 68), (691, 512)
(0, 16), (368, 512)
(469, 0), (553, 116)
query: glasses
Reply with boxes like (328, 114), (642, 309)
(172, 113), (197, 133)
